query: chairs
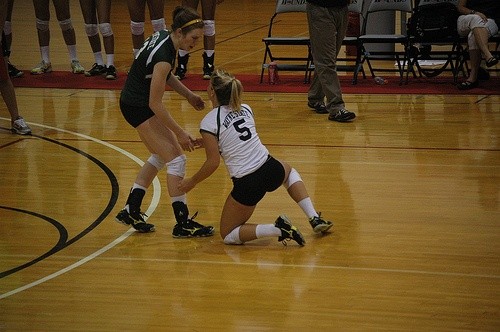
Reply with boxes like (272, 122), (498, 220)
(260, 0), (500, 85)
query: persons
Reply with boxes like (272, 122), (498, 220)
(307, 0), (357, 122)
(32, 0), (85, 75)
(456, 0), (500, 90)
(0, 0), (31, 135)
(129, 0), (166, 59)
(115, 5), (214, 238)
(178, 69), (334, 247)
(79, 1), (117, 79)
(3, 0), (24, 78)
(416, 0), (457, 58)
(174, 0), (224, 79)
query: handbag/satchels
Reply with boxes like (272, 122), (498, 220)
(407, 2), (459, 43)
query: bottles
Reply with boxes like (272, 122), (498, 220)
(374, 77), (388, 84)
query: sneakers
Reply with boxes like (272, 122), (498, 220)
(71, 60), (85, 73)
(307, 98), (329, 114)
(457, 79), (482, 90)
(115, 207), (155, 232)
(84, 63), (107, 76)
(308, 212), (334, 233)
(8, 60), (24, 77)
(486, 57), (498, 67)
(172, 219), (215, 238)
(274, 214), (306, 246)
(30, 60), (52, 74)
(202, 62), (214, 79)
(106, 65), (117, 80)
(328, 108), (356, 121)
(11, 116), (32, 135)
(174, 64), (187, 79)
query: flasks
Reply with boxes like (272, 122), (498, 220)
(268, 62), (278, 85)
